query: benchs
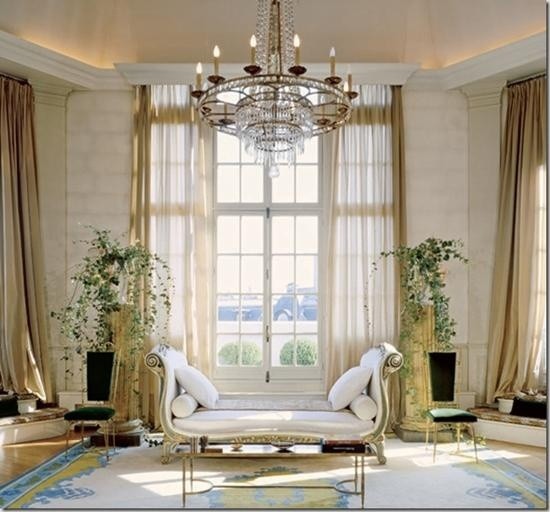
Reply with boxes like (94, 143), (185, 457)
(143, 341), (404, 465)
(467, 406), (547, 448)
(0, 408), (70, 445)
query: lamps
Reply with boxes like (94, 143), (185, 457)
(189, 1), (358, 179)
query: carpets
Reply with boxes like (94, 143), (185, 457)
(0, 429), (548, 510)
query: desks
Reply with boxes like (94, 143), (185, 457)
(170, 441), (377, 510)
(57, 389), (105, 428)
(455, 391), (478, 412)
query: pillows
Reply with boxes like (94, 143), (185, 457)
(171, 391), (199, 418)
(511, 399), (547, 420)
(0, 397), (21, 418)
(174, 365), (220, 409)
(349, 392), (377, 420)
(328, 365), (372, 410)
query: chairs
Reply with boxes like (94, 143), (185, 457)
(422, 349), (480, 467)
(62, 340), (124, 465)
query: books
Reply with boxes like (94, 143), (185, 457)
(320, 439), (365, 454)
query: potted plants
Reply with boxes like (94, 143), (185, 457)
(364, 235), (487, 446)
(50, 222), (176, 447)
